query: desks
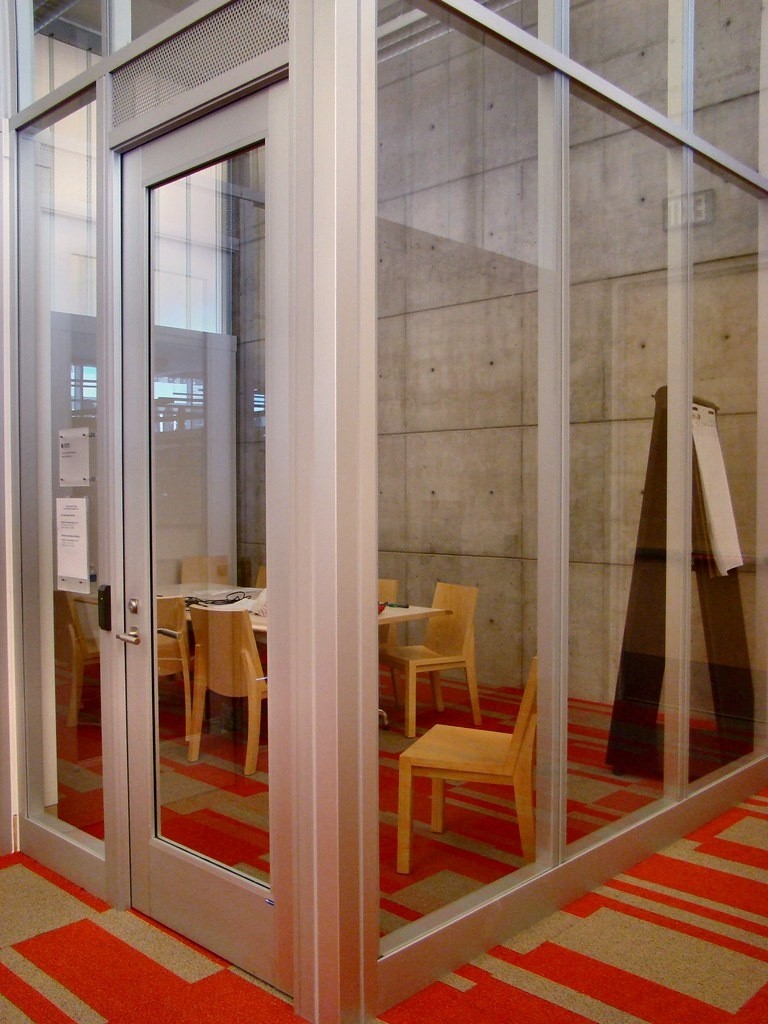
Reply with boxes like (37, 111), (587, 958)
(155, 583), (454, 731)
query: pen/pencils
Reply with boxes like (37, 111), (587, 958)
(387, 603), (409, 608)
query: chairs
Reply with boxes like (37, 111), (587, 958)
(376, 580), (397, 704)
(156, 597), (194, 741)
(53, 591), (100, 727)
(180, 555), (227, 584)
(379, 582), (480, 737)
(186, 603), (269, 774)
(396, 656), (540, 875)
